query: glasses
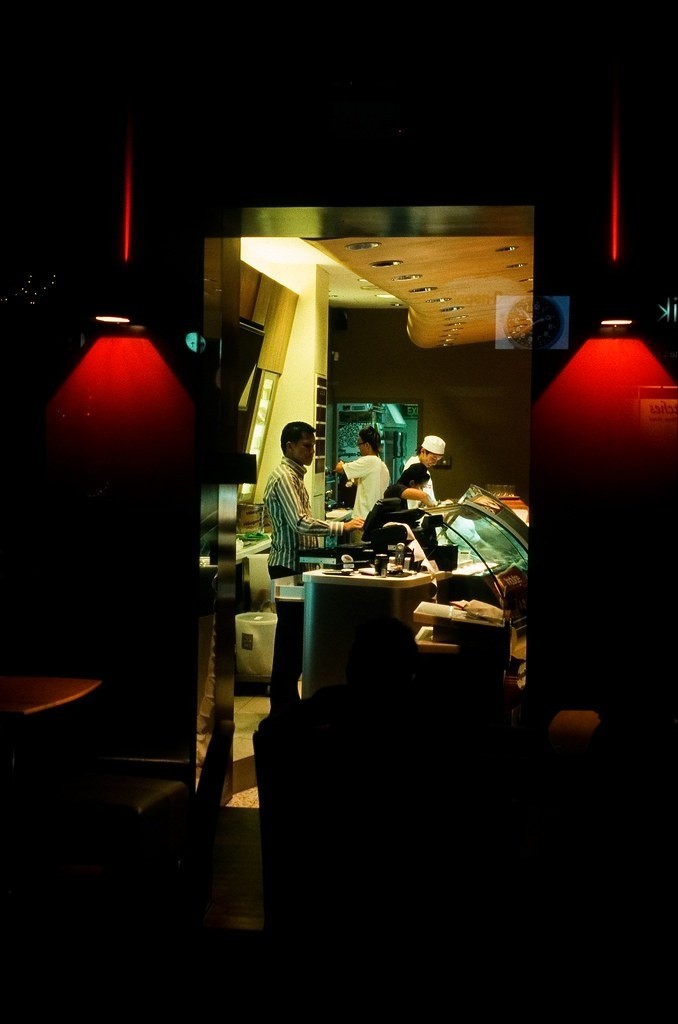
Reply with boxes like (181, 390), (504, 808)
(357, 442), (365, 446)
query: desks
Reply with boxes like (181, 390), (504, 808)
(0, 676), (104, 716)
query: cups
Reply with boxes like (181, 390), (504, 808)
(487, 485), (515, 496)
(375, 554), (387, 578)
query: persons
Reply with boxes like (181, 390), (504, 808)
(404, 435), (447, 509)
(335, 426), (391, 544)
(384, 461), (435, 514)
(263, 422), (366, 710)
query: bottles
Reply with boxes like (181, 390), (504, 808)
(396, 543), (404, 566)
(325, 497), (332, 511)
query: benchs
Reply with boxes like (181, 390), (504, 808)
(84, 777), (189, 833)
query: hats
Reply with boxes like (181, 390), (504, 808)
(422, 436), (445, 454)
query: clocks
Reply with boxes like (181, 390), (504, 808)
(504, 296), (565, 350)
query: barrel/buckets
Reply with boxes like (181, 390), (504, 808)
(236, 612), (277, 675)
